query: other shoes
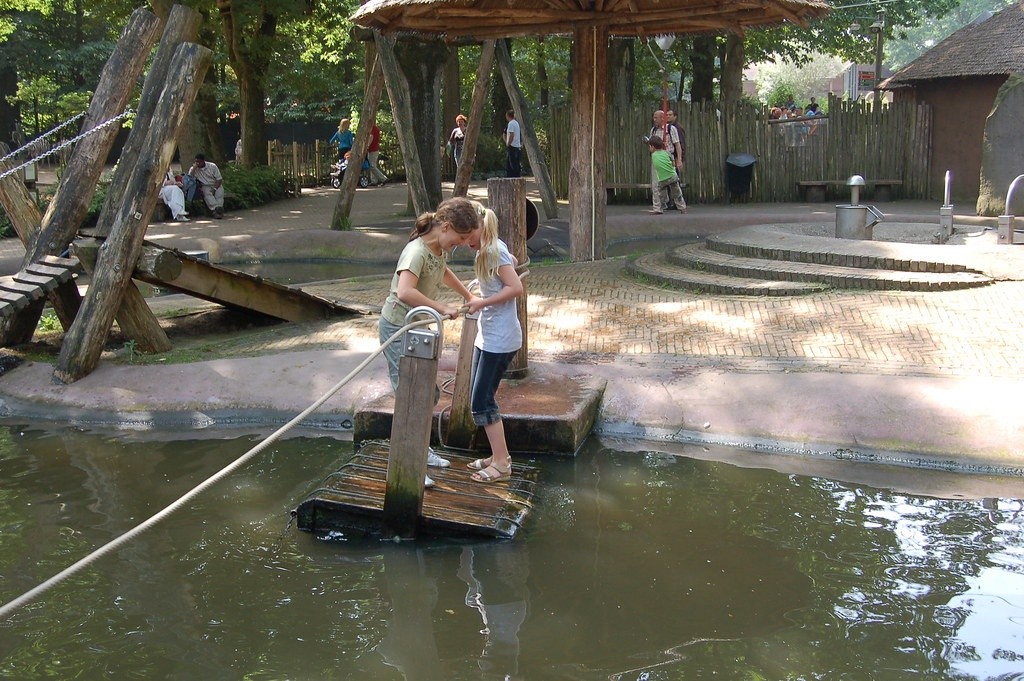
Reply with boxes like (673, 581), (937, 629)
(426, 447), (450, 467)
(178, 211), (188, 215)
(214, 206), (224, 219)
(424, 474), (434, 486)
(177, 216), (190, 221)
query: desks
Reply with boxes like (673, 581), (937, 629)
(150, 193), (236, 222)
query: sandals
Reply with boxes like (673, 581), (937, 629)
(470, 463), (511, 483)
(467, 455), (512, 470)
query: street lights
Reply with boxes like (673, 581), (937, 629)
(655, 33), (676, 209)
(850, 17), (884, 116)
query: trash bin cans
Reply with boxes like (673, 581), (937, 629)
(726, 153), (756, 202)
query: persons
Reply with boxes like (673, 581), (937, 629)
(463, 199), (524, 483)
(188, 153), (225, 219)
(330, 121), (388, 187)
(445, 115), (476, 168)
(642, 110), (688, 216)
(379, 197), (482, 488)
(503, 110), (521, 178)
(158, 167), (190, 221)
(761, 95), (825, 146)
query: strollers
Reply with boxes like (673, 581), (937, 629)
(330, 154), (369, 189)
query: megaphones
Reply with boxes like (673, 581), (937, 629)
(869, 22), (883, 33)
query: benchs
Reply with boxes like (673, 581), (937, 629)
(605, 184), (689, 205)
(798, 179), (902, 203)
